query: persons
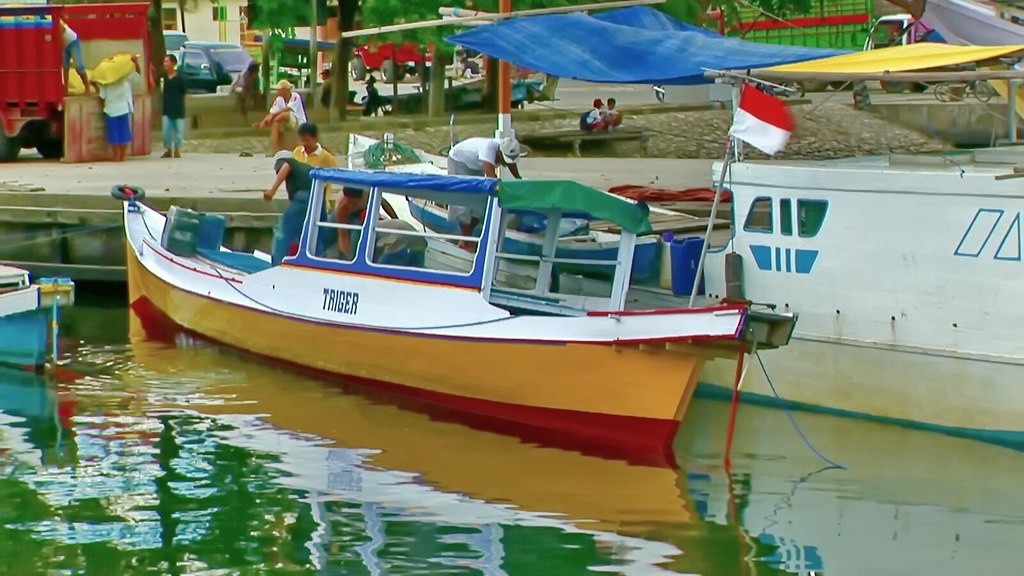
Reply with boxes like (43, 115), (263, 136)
(159, 54), (187, 160)
(252, 78), (309, 152)
(317, 70), (333, 106)
(582, 99), (606, 134)
(445, 136), (523, 254)
(57, 20), (92, 96)
(605, 97), (623, 132)
(328, 179), (399, 259)
(262, 147), (330, 269)
(236, 62), (267, 120)
(290, 123), (340, 195)
(90, 54), (142, 163)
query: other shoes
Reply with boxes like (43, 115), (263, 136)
(239, 151), (252, 156)
(174, 153), (180, 157)
(161, 153), (171, 157)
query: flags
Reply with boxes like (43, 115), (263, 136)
(726, 83), (796, 155)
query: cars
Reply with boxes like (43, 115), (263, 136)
(351, 33), (452, 84)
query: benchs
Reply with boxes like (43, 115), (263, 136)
(558, 235), (594, 241)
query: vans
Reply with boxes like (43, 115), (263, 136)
(163, 30), (253, 93)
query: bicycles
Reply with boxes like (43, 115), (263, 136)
(934, 63), (994, 102)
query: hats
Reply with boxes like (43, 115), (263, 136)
(319, 69), (330, 74)
(274, 149), (294, 160)
(460, 57), (467, 63)
(594, 99), (605, 107)
(273, 79), (291, 89)
(250, 60), (262, 67)
(500, 137), (520, 163)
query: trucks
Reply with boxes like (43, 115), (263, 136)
(864, 14), (947, 93)
(698, 0), (903, 91)
(0, 0), (151, 163)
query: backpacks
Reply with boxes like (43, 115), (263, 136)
(580, 109), (596, 130)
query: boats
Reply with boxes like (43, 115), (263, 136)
(122, 68), (1024, 469)
(362, 79), (542, 114)
(0, 264), (75, 367)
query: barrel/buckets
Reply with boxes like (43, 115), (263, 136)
(197, 212), (226, 251)
(658, 232), (675, 290)
(670, 235), (711, 296)
(160, 204), (200, 258)
